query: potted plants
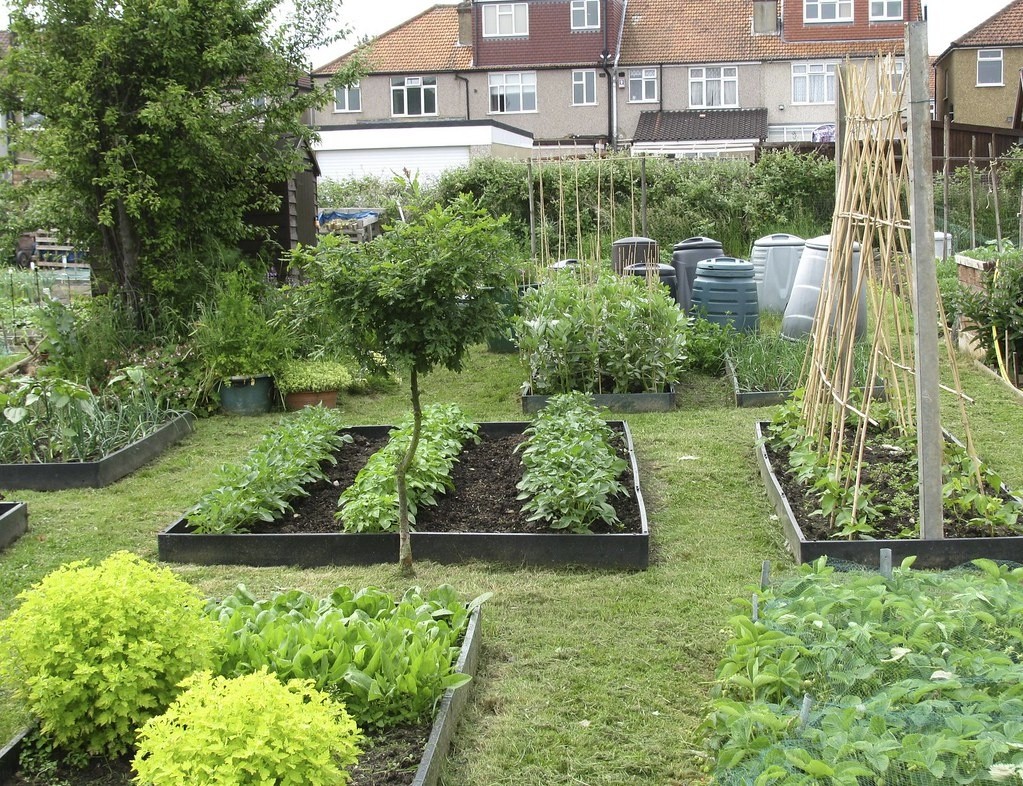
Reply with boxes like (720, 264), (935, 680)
(189, 263), (353, 416)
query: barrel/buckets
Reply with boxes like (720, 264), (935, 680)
(690, 257), (760, 343)
(625, 261), (678, 303)
(670, 237), (724, 317)
(777, 237), (867, 346)
(934, 232), (952, 259)
(610, 237), (658, 272)
(750, 234), (805, 315)
